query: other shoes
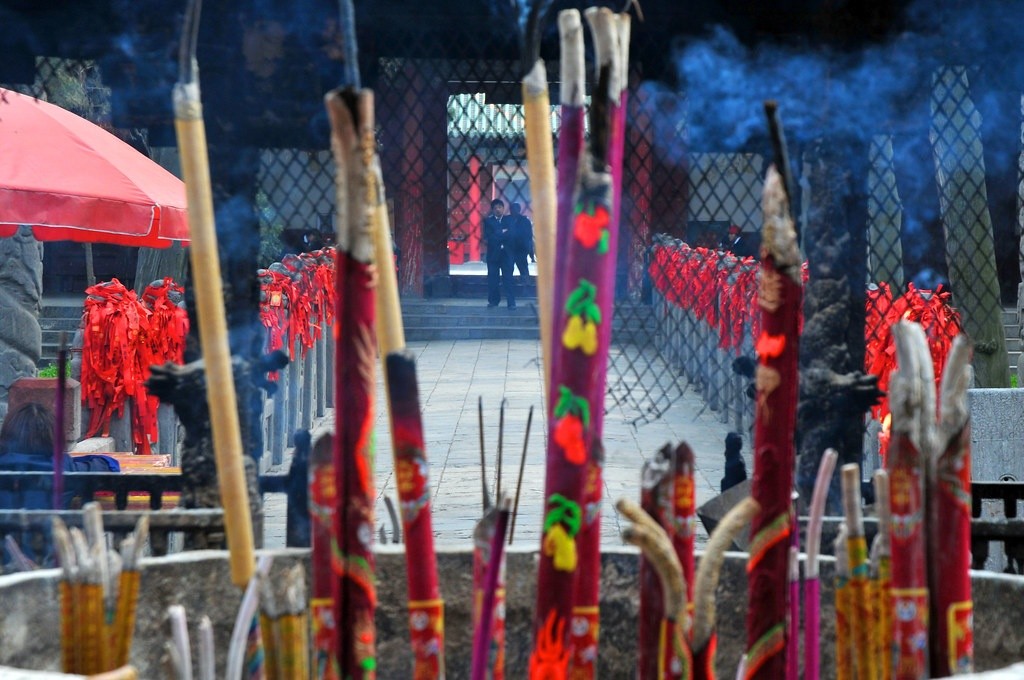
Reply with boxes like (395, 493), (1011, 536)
(525, 279), (530, 286)
(487, 302), (497, 307)
(508, 304), (517, 311)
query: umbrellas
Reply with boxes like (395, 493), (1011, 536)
(0, 86), (191, 250)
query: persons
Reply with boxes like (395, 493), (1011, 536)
(298, 228), (322, 255)
(483, 199), (516, 310)
(0, 402), (121, 574)
(500, 203), (533, 288)
(720, 224), (747, 257)
(522, 215), (535, 262)
